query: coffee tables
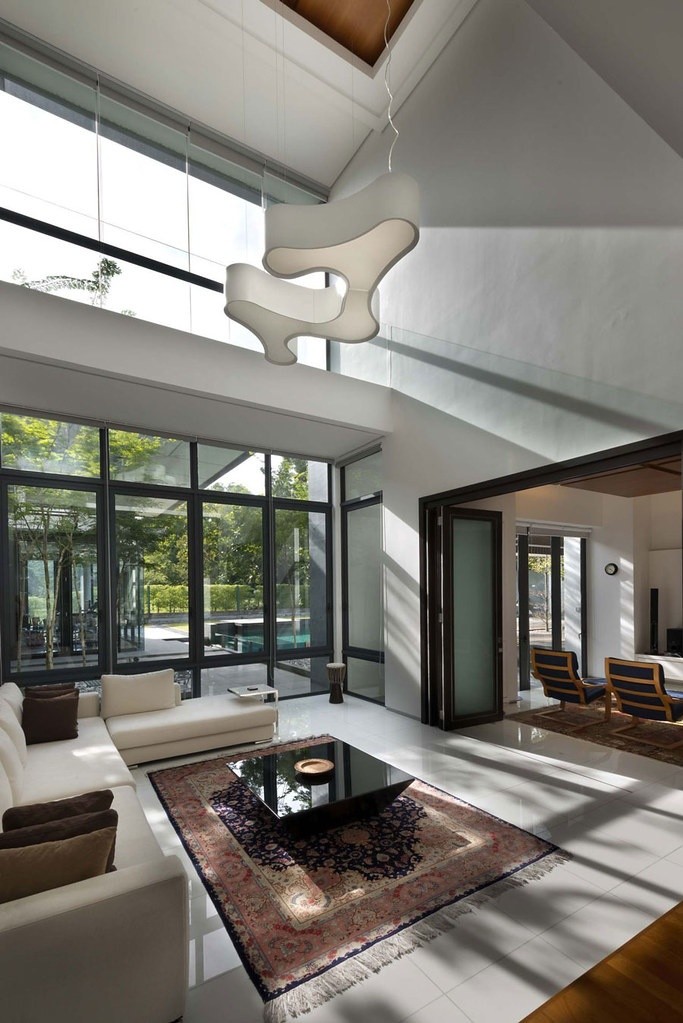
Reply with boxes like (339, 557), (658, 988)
(226, 740), (415, 829)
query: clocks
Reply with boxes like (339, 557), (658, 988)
(605, 563), (618, 575)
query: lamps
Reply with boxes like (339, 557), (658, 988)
(221, 0), (423, 365)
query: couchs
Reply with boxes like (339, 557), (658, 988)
(1, 683), (277, 1023)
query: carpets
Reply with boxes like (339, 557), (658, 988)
(146, 734), (572, 1023)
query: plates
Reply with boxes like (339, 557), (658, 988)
(294, 759), (334, 774)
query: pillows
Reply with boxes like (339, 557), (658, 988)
(22, 683), (79, 744)
(0, 789), (118, 904)
(100, 668), (175, 718)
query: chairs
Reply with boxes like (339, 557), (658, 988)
(532, 647), (611, 728)
(604, 657), (683, 748)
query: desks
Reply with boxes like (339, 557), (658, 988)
(227, 684), (279, 744)
